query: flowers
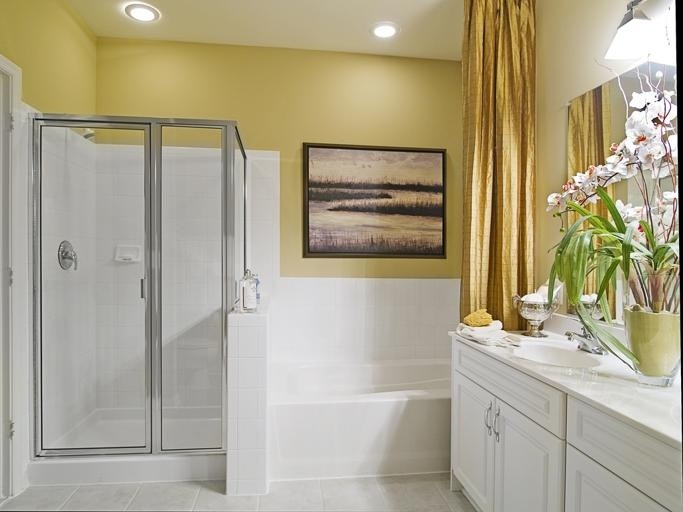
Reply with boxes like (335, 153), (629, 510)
(545, 63), (680, 367)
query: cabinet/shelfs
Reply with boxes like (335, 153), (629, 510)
(563, 396), (682, 511)
(449, 330), (566, 510)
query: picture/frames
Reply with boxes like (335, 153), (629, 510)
(301, 143), (446, 259)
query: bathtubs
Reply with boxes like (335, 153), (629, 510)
(271, 357), (451, 483)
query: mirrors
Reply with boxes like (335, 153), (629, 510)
(565, 55), (681, 327)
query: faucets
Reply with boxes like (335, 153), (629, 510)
(564, 324), (608, 355)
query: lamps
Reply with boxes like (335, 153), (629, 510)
(603, 1), (661, 61)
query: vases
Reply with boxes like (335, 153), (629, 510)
(620, 301), (681, 387)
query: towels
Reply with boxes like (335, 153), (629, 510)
(456, 321), (522, 348)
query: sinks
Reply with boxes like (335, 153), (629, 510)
(510, 340), (602, 371)
